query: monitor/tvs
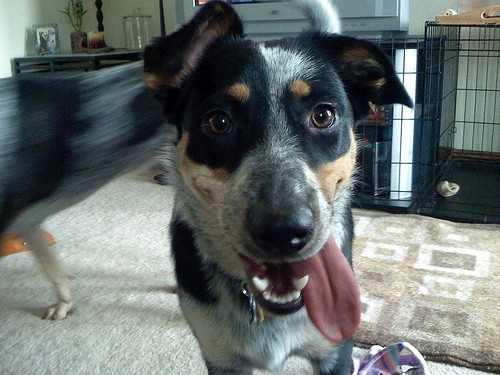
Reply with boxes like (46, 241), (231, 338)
(175, 0), (410, 40)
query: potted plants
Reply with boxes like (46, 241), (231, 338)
(59, 0), (88, 53)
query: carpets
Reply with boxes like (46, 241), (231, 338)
(348, 208), (500, 373)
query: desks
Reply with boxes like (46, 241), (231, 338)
(12, 48), (143, 75)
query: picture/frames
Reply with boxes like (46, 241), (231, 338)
(33, 24), (60, 55)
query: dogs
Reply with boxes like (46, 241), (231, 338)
(142, 0), (415, 375)
(0, 59), (176, 320)
